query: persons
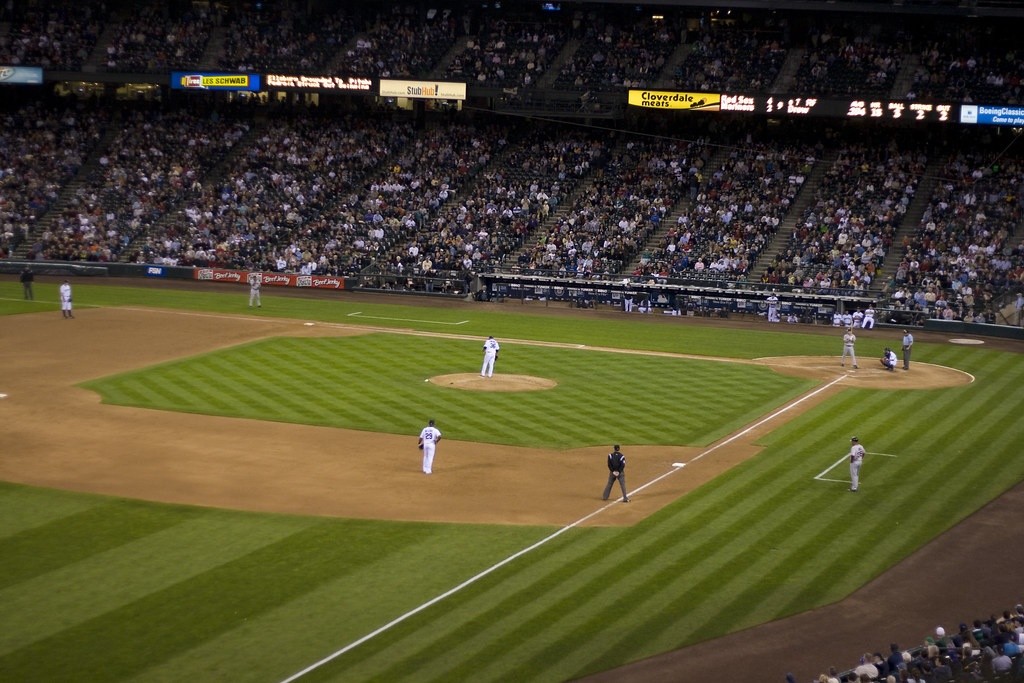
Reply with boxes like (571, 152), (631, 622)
(842, 328), (913, 371)
(0, 0), (1024, 329)
(847, 436), (866, 492)
(602, 445), (631, 502)
(418, 420), (441, 474)
(480, 336), (500, 378)
(786, 604), (1024, 683)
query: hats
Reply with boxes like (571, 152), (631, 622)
(614, 445), (620, 448)
(925, 637), (934, 644)
(937, 627), (945, 635)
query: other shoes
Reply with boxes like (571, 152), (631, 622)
(841, 363), (844, 367)
(847, 488), (856, 492)
(853, 365), (857, 369)
(623, 500), (631, 502)
(902, 367), (909, 370)
(889, 369), (893, 372)
(603, 498), (608, 500)
(885, 367), (890, 370)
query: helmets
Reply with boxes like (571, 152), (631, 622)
(847, 328), (852, 331)
(852, 437), (859, 442)
(902, 329), (909, 333)
